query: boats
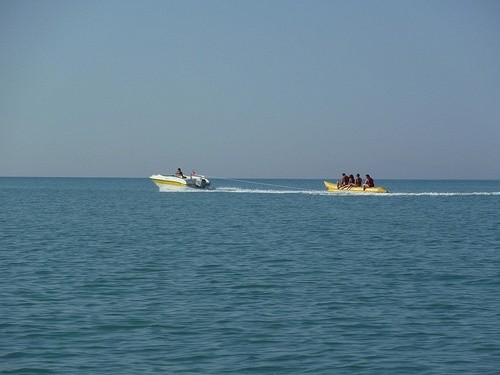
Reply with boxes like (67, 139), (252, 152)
(324, 181), (388, 193)
(148, 173), (210, 192)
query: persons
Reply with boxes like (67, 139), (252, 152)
(362, 174), (374, 191)
(337, 173), (362, 190)
(175, 167), (187, 179)
(191, 170), (196, 176)
